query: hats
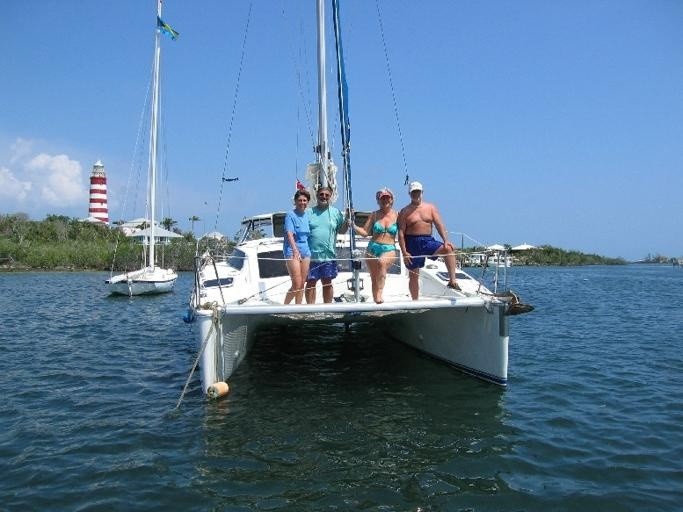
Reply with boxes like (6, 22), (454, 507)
(408, 180), (423, 194)
(377, 189), (394, 210)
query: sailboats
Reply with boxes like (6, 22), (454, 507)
(184, 1), (533, 398)
(105, 0), (182, 297)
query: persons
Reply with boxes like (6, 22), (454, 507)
(304, 187), (354, 304)
(346, 187), (400, 304)
(396, 181), (462, 300)
(281, 190), (310, 305)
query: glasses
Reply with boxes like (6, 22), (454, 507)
(319, 193), (332, 198)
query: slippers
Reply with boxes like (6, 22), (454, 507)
(447, 282), (462, 291)
